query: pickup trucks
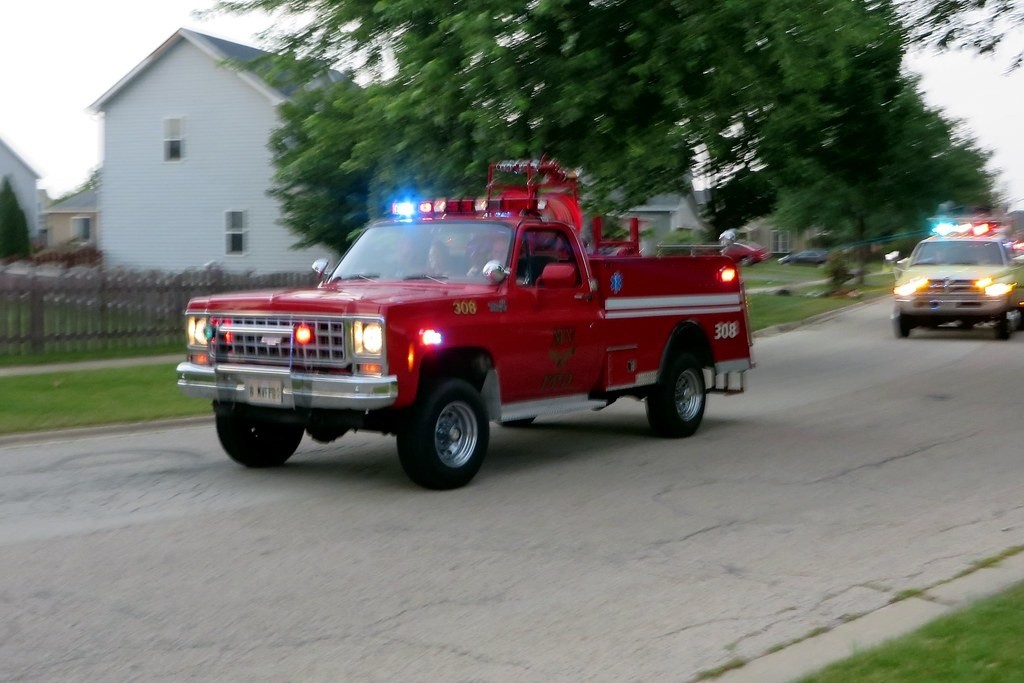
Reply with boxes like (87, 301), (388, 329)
(175, 158), (756, 488)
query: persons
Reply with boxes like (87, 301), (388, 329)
(428, 235), (524, 285)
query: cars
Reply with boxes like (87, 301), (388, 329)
(776, 248), (829, 266)
(885, 217), (1024, 339)
(720, 239), (772, 267)
